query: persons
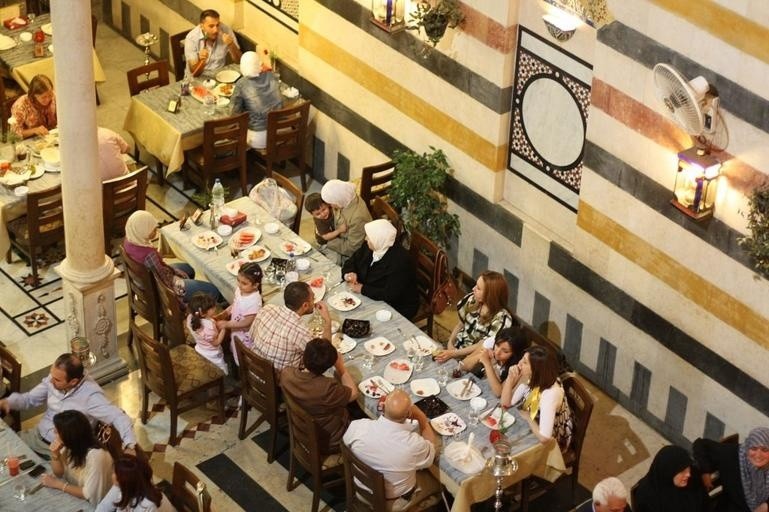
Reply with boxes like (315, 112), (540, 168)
(312, 180), (372, 266)
(11, 74), (56, 138)
(630, 445), (715, 512)
(1, 355), (135, 463)
(695, 428), (768, 511)
(247, 283), (331, 409)
(184, 8), (243, 79)
(94, 451), (176, 512)
(305, 193), (346, 247)
(40, 411), (115, 504)
(342, 220), (420, 317)
(126, 209), (222, 306)
(571, 476), (634, 512)
(433, 271), (511, 362)
(461, 327), (524, 395)
(280, 339), (358, 454)
(342, 392), (443, 511)
(502, 348), (572, 452)
(97, 125), (135, 189)
(186, 293), (225, 412)
(213, 264), (266, 406)
(231, 52), (285, 152)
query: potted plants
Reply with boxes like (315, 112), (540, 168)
(404, 0), (467, 47)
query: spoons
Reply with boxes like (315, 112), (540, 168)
(464, 433), (475, 465)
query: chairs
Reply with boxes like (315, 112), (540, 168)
(248, 160), (305, 236)
(407, 223), (444, 338)
(118, 243), (174, 346)
(693, 433), (740, 512)
(0, 63), (26, 144)
(521, 324), (563, 364)
(171, 27), (195, 82)
(0, 4), (20, 25)
(349, 161), (396, 221)
(97, 420), (123, 462)
(6, 183), (64, 287)
(248, 100), (311, 192)
(281, 385), (344, 511)
(522, 376), (594, 512)
(370, 195), (400, 230)
(2, 94), (21, 143)
(183, 111), (249, 197)
(102, 165), (148, 258)
(1, 345), (25, 438)
(232, 335), (290, 463)
(91, 14), (100, 106)
(129, 319), (227, 446)
(170, 461), (212, 512)
(127, 60), (169, 97)
(340, 441), (385, 511)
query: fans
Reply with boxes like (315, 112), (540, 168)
(652, 63), (730, 155)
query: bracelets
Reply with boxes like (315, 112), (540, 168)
(60, 482), (73, 493)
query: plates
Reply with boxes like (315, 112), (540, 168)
(376, 310), (392, 322)
(469, 397), (487, 410)
(342, 318), (370, 338)
(20, 32), (33, 42)
(364, 336), (396, 356)
(191, 231), (223, 248)
(280, 236), (311, 256)
(326, 291), (362, 312)
(273, 257), (287, 266)
(41, 23), (52, 34)
(430, 412), (467, 436)
(265, 222), (279, 233)
(216, 70), (241, 82)
(14, 186), (28, 196)
(240, 244), (273, 263)
(295, 259), (311, 270)
(357, 376), (394, 399)
(228, 227), (262, 250)
(383, 359), (414, 385)
(45, 169), (60, 172)
(286, 272), (298, 283)
(402, 335), (438, 356)
(331, 332), (357, 354)
(216, 97), (231, 107)
(305, 280), (326, 304)
(413, 394), (450, 420)
(29, 167), (45, 179)
(478, 408), (516, 431)
(218, 225), (233, 236)
(213, 84), (235, 96)
(48, 128), (57, 135)
(203, 79), (216, 87)
(410, 378), (441, 398)
(191, 89), (214, 103)
(445, 378), (482, 400)
(1, 35), (17, 51)
(225, 258), (244, 276)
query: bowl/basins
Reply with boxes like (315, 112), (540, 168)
(444, 441), (485, 474)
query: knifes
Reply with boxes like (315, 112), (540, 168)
(482, 402), (500, 420)
(460, 377), (473, 398)
(370, 379), (388, 396)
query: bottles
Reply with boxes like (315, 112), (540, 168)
(204, 79), (215, 116)
(32, 25), (45, 58)
(181, 80), (189, 96)
(211, 177), (225, 218)
(7, 117), (25, 159)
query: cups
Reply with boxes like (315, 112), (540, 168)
(399, 382), (404, 391)
(8, 458), (19, 476)
(438, 369), (448, 387)
(16, 147), (28, 162)
(28, 13), (35, 22)
(263, 262), (275, 281)
(469, 407), (479, 426)
(412, 352), (425, 372)
(406, 344), (417, 363)
(361, 353), (374, 370)
(40, 149), (59, 163)
(453, 428), (466, 442)
(203, 96), (215, 115)
(13, 484), (25, 501)
(276, 266), (285, 285)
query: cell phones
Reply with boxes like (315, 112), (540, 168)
(19, 460), (35, 471)
(28, 465), (46, 478)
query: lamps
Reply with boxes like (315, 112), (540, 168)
(669, 146), (727, 223)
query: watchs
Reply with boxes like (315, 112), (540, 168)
(126, 445), (136, 451)
